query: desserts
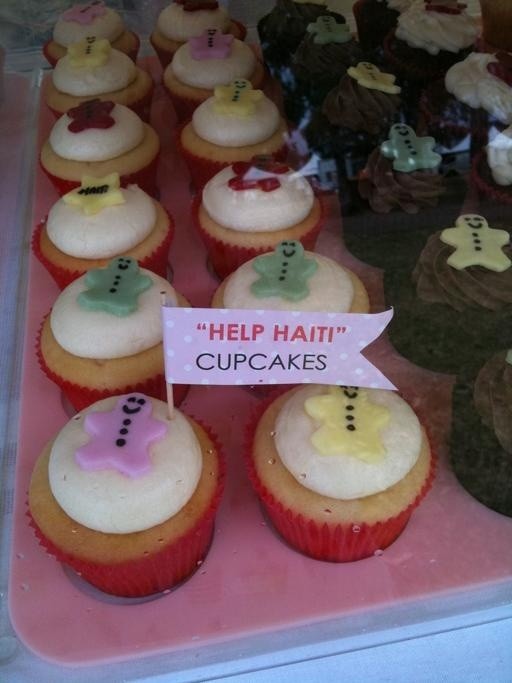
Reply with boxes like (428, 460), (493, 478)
(25, 0), (512, 599)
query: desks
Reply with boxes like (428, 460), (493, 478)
(0, 14), (511, 682)
